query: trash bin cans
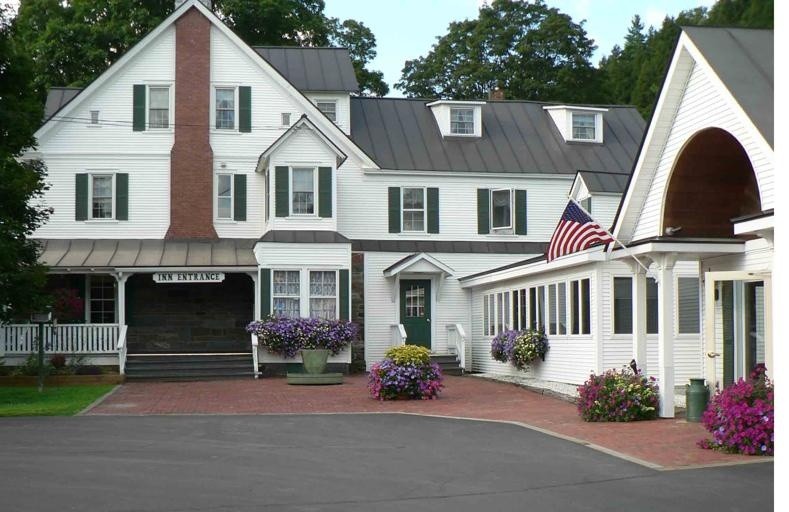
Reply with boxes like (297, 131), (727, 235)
(687, 379), (707, 422)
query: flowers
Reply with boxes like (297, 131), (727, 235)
(698, 363), (773, 459)
(576, 359), (661, 422)
(490, 326), (550, 369)
(247, 315), (358, 357)
(368, 343), (444, 402)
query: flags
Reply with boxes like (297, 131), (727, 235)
(547, 199), (615, 265)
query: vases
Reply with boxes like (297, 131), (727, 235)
(301, 349), (331, 375)
